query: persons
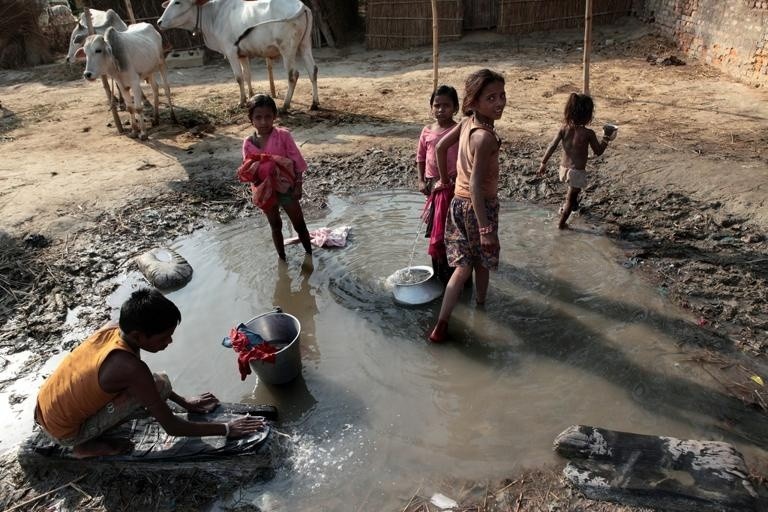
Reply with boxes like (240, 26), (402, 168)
(32, 287), (264, 460)
(242, 95), (313, 273)
(429, 69), (506, 344)
(416, 84), (474, 288)
(533, 93), (618, 229)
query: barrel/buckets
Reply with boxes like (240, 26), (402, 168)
(238, 309), (302, 386)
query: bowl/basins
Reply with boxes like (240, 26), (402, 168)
(387, 265), (445, 304)
(602, 125), (619, 140)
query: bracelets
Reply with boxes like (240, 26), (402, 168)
(601, 140), (608, 146)
(603, 135), (610, 140)
(221, 422), (229, 437)
(479, 224), (494, 235)
(439, 178), (453, 188)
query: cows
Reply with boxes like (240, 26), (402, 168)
(65, 8), (152, 111)
(157, 0), (321, 114)
(73, 22), (177, 141)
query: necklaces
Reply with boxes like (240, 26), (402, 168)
(480, 121), (495, 130)
